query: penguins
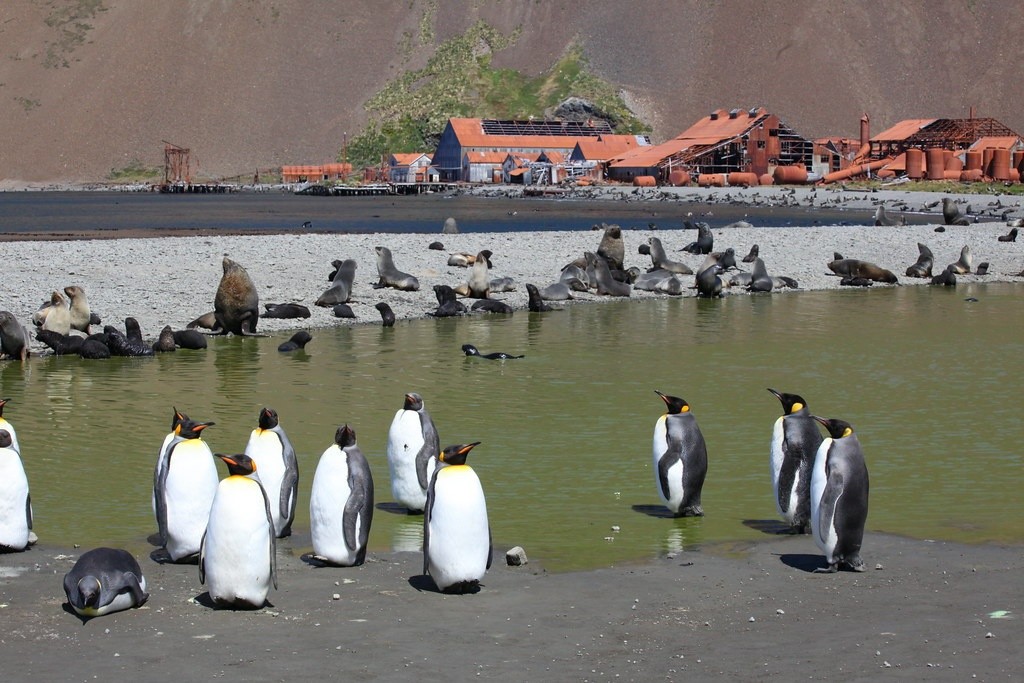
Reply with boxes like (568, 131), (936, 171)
(388, 392), (437, 510)
(150, 408), (192, 520)
(63, 548), (148, 616)
(423, 440), (491, 595)
(765, 385), (827, 534)
(808, 412), (869, 576)
(155, 418), (219, 564)
(304, 423), (373, 569)
(0, 397), (20, 457)
(198, 449), (277, 614)
(652, 391), (706, 518)
(243, 407), (298, 539)
(1, 430), (33, 555)
